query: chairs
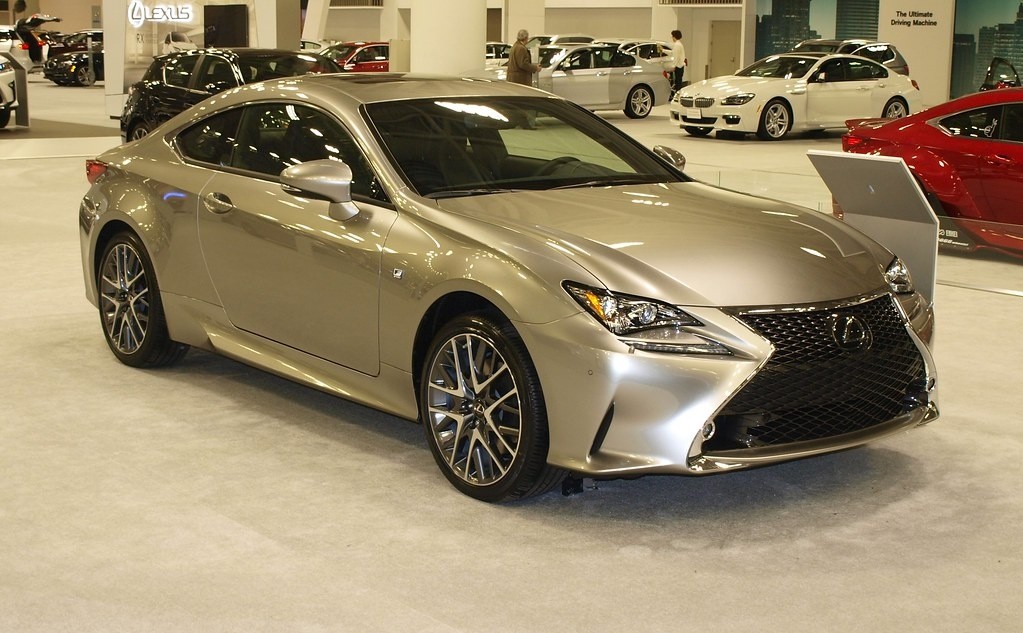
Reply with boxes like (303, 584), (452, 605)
(436, 122), (500, 190)
(213, 63), (232, 83)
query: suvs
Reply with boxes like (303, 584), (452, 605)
(788, 39), (909, 76)
(0, 13), (63, 75)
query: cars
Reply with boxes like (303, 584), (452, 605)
(42, 52), (105, 87)
(842, 87), (1023, 259)
(489, 44), (672, 120)
(0, 51), (18, 129)
(38, 28), (103, 59)
(76, 73), (942, 507)
(308, 42), (390, 72)
(300, 39), (332, 54)
(120, 47), (349, 144)
(669, 52), (921, 141)
(527, 35), (595, 47)
(486, 41), (513, 66)
(592, 38), (688, 85)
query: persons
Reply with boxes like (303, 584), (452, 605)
(505, 30), (541, 131)
(659, 30), (686, 92)
